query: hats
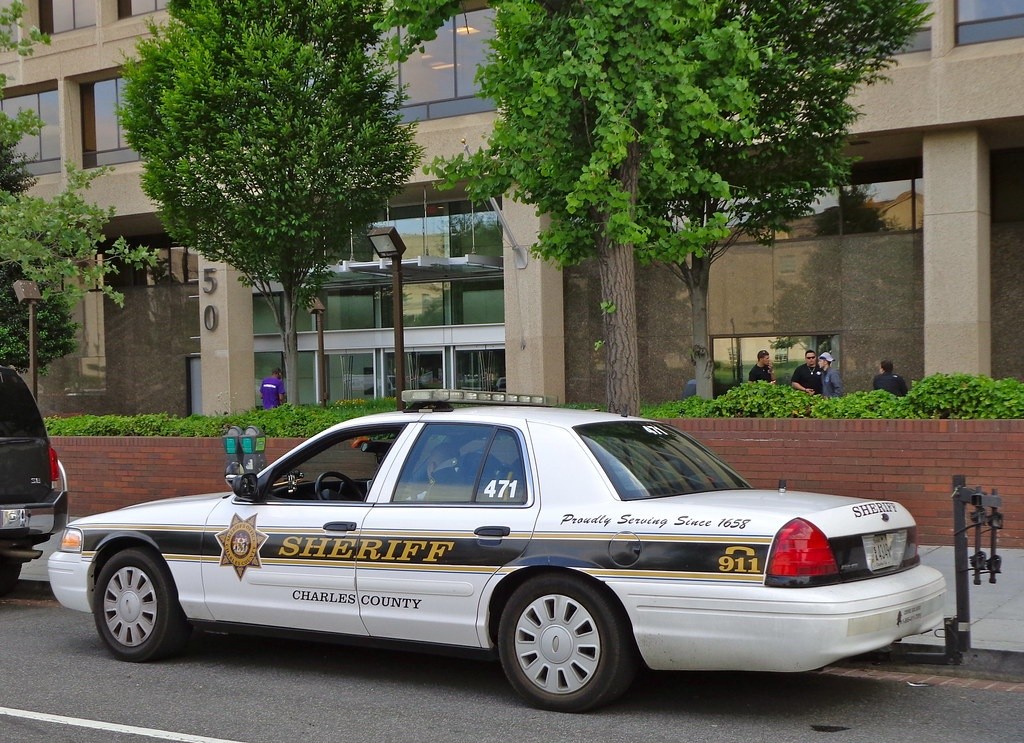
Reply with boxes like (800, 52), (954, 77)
(819, 352), (835, 363)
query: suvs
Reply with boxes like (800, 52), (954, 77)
(0, 365), (71, 600)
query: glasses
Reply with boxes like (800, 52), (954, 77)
(805, 356), (816, 360)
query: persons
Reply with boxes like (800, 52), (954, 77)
(406, 442), (460, 502)
(260, 367), (285, 409)
(791, 350), (823, 394)
(749, 350), (776, 383)
(818, 352), (842, 398)
(873, 360), (908, 398)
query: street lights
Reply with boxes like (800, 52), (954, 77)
(306, 296), (327, 407)
(368, 227), (407, 412)
(14, 280), (45, 400)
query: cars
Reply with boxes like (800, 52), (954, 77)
(46, 388), (946, 713)
